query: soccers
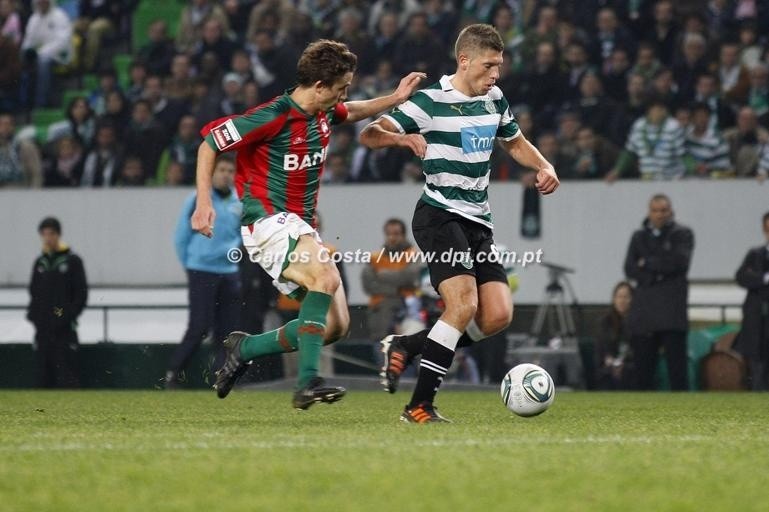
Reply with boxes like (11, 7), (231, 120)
(500, 364), (554, 416)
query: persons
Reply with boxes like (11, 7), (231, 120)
(1, 0), (769, 182)
(360, 218), (428, 377)
(189, 37), (427, 408)
(26, 218), (87, 389)
(600, 282), (634, 389)
(358, 22), (560, 426)
(730, 211), (768, 393)
(624, 195), (694, 391)
(162, 153), (244, 392)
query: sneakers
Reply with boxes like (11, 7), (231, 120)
(211, 330), (254, 400)
(292, 375), (347, 412)
(153, 368), (186, 390)
(379, 332), (414, 395)
(399, 399), (454, 428)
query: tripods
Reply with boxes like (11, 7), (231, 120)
(528, 258), (580, 346)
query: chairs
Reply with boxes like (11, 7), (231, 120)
(644, 321), (746, 393)
